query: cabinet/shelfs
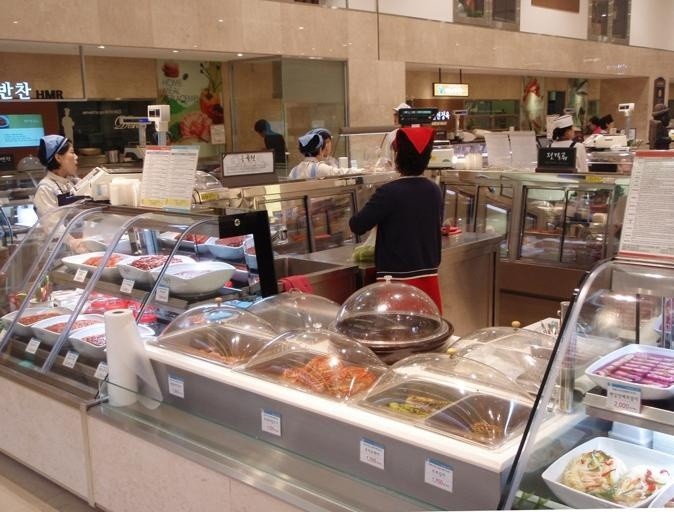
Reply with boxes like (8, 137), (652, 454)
(0, 163), (674, 512)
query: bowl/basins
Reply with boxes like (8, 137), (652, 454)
(78, 147), (102, 155)
(540, 434), (673, 508)
(155, 227), (259, 272)
(76, 236), (134, 255)
(583, 343), (674, 400)
(1, 249), (236, 360)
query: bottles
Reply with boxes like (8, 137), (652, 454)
(469, 142), (482, 170)
(567, 195), (589, 220)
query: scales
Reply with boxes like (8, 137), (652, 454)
(452, 110), (468, 136)
(112, 117), (152, 162)
(148, 104), (171, 146)
(618, 103), (635, 146)
(396, 105), (456, 170)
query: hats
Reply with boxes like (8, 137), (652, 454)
(553, 115), (573, 128)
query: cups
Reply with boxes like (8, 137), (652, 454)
(127, 226), (156, 256)
(104, 149), (119, 164)
(338, 156), (349, 168)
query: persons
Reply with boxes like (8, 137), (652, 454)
(33, 134), (87, 273)
(349, 127), (444, 321)
(546, 115), (588, 173)
(603, 115), (614, 133)
(380, 102), (413, 171)
(255, 119), (288, 163)
(305, 128), (339, 169)
(288, 134), (367, 180)
(589, 117), (608, 134)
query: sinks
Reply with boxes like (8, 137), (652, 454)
(272, 256), (344, 281)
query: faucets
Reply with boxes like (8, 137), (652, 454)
(272, 229), (288, 242)
